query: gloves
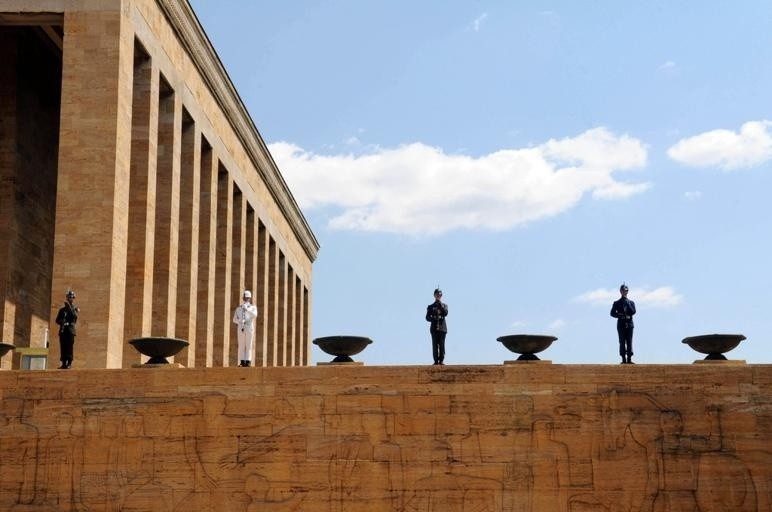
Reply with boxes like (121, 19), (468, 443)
(242, 304), (248, 311)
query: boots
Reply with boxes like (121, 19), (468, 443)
(240, 360), (250, 367)
(58, 361), (72, 369)
(433, 360), (445, 365)
(621, 358), (634, 364)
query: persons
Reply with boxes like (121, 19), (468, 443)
(56, 289), (78, 369)
(426, 289), (448, 366)
(232, 290), (257, 367)
(610, 285), (637, 365)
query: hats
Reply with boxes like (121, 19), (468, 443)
(244, 290), (251, 298)
(620, 285), (628, 291)
(66, 291), (74, 298)
(434, 289), (443, 296)
